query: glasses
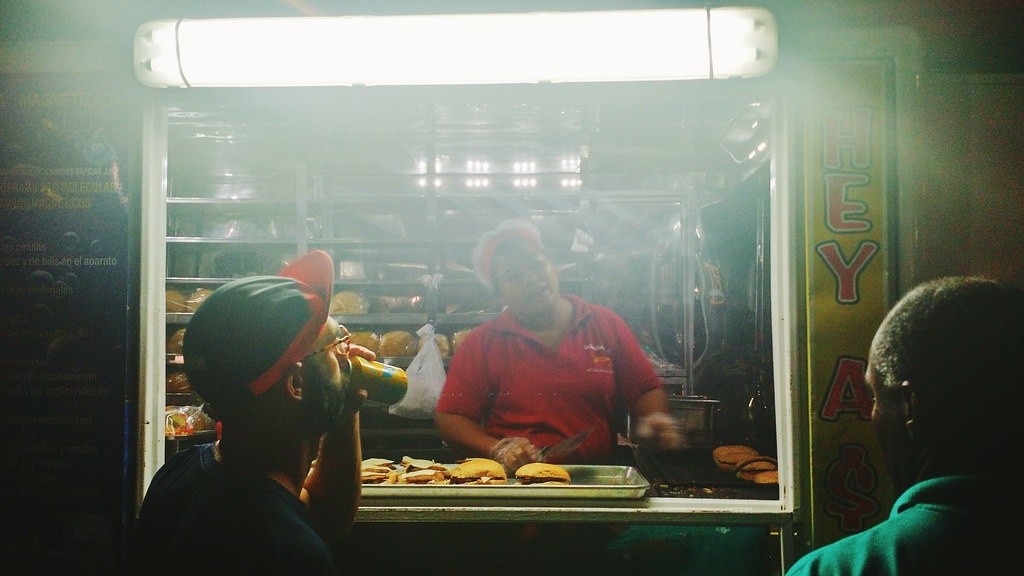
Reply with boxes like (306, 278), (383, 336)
(298, 325), (354, 362)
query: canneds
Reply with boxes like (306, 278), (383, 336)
(339, 356), (408, 405)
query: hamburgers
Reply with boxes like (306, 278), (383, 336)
(359, 455), (570, 485)
(712, 445), (779, 489)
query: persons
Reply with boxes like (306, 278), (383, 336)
(784, 279), (1024, 576)
(119, 252), (378, 576)
(435, 218), (679, 471)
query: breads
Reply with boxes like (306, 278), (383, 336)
(330, 290), (476, 358)
(165, 287), (214, 432)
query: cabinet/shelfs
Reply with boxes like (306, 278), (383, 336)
(160, 188), (697, 400)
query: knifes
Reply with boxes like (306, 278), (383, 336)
(535, 425), (589, 462)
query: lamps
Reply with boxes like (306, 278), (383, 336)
(132, 6), (778, 90)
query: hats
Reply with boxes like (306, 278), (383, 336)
(472, 218), (546, 293)
(181, 248), (336, 421)
